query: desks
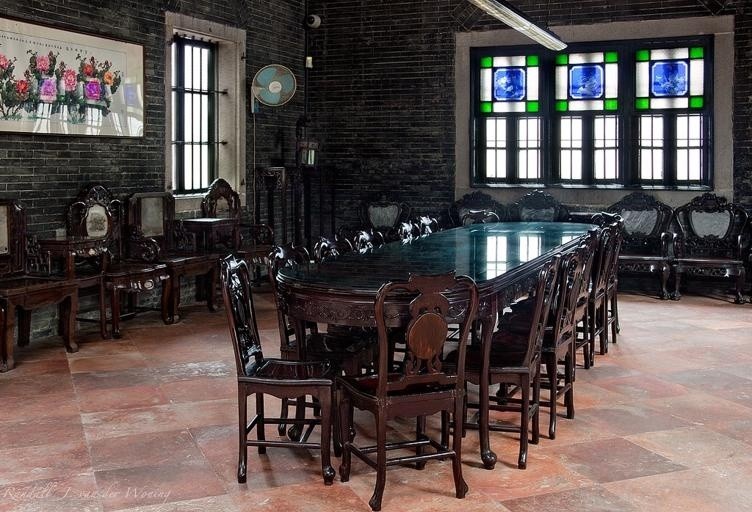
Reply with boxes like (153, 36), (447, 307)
(276, 223), (602, 469)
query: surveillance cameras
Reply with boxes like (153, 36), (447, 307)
(306, 13), (320, 27)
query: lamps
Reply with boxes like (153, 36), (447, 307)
(469, 0), (568, 51)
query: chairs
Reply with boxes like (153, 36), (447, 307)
(313, 190), (624, 352)
(219, 252), (336, 486)
(440, 252), (563, 470)
(672, 190), (750, 306)
(200, 179), (276, 307)
(491, 244), (592, 440)
(66, 184), (174, 339)
(124, 191), (218, 326)
(1, 190), (79, 371)
(599, 191), (675, 300)
(335, 269), (481, 511)
(266, 243), (376, 442)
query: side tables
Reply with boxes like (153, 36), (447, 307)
(35, 235), (111, 342)
(184, 217), (242, 310)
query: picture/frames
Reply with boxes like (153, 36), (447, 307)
(0, 13), (147, 140)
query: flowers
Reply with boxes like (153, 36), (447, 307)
(85, 81), (100, 100)
(103, 71), (113, 84)
(64, 69), (77, 87)
(0, 54), (8, 69)
(83, 64), (93, 76)
(16, 80), (28, 93)
(36, 56), (48, 73)
(40, 80), (55, 97)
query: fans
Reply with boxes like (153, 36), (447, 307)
(251, 64), (296, 113)
